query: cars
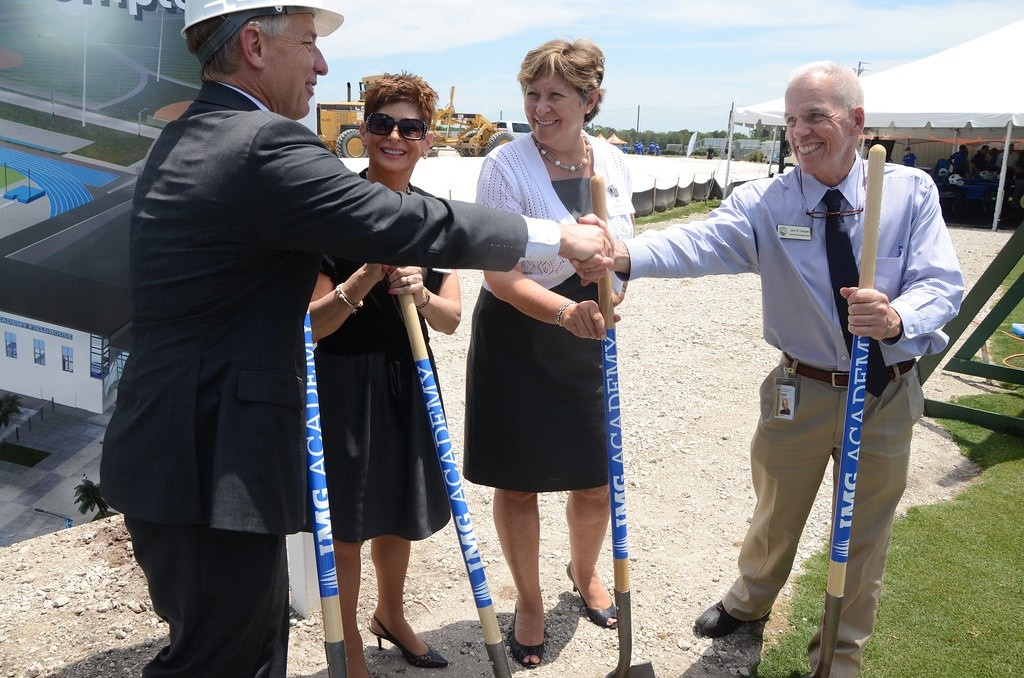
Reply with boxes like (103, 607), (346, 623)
(493, 121), (532, 139)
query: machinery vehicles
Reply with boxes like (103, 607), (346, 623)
(316, 75), (515, 158)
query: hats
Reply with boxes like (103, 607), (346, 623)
(904, 147), (911, 151)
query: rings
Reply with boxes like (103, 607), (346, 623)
(407, 276), (411, 284)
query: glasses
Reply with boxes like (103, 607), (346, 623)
(801, 164), (867, 218)
(365, 112), (428, 140)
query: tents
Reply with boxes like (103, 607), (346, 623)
(597, 133), (627, 144)
(723, 19), (1024, 231)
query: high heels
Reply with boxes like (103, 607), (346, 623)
(567, 560), (618, 629)
(368, 612), (448, 668)
(511, 601), (544, 670)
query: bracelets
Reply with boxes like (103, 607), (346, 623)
(416, 286), (430, 310)
(336, 283), (363, 308)
(555, 301), (576, 325)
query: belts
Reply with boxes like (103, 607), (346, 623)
(780, 352), (916, 389)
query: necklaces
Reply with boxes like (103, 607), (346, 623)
(536, 134), (591, 171)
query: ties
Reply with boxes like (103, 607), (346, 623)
(820, 189), (890, 398)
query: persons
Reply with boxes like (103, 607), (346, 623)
(949, 145), (1024, 179)
(634, 141), (644, 155)
(99, 0), (615, 678)
(570, 60), (964, 678)
(780, 399), (790, 415)
(706, 145), (714, 159)
(622, 144), (627, 153)
(649, 141), (660, 156)
(309, 74), (462, 678)
(463, 39), (636, 668)
(903, 147), (917, 166)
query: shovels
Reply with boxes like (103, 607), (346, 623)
(590, 176), (656, 678)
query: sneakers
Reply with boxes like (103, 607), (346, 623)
(696, 600), (770, 638)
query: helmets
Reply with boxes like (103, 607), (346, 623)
(981, 171), (992, 181)
(948, 174), (964, 186)
(181, 0), (344, 38)
(939, 168), (948, 177)
